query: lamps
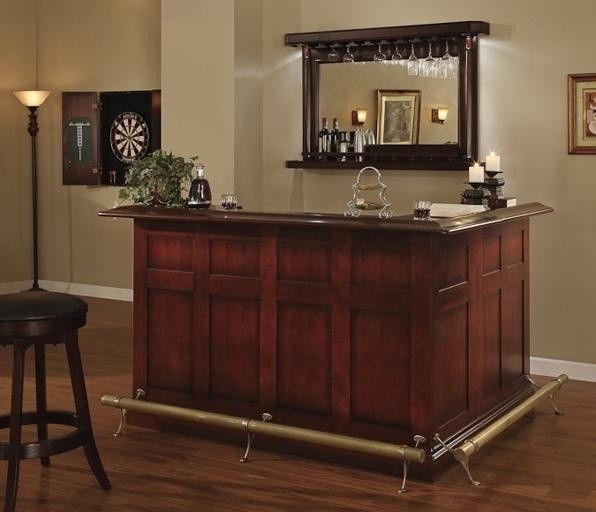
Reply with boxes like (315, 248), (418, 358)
(14, 89), (52, 293)
(352, 108), (366, 123)
(432, 107), (447, 123)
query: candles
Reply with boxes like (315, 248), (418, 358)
(468, 163), (485, 181)
(484, 151), (501, 173)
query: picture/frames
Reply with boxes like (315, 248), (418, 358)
(376, 87), (421, 146)
(568, 73), (596, 155)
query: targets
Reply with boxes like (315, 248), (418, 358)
(110, 112), (149, 163)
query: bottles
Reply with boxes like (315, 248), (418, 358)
(185, 163), (212, 209)
(336, 130), (350, 162)
(353, 128), (366, 162)
(328, 117), (340, 143)
(317, 117), (331, 159)
(363, 129), (375, 145)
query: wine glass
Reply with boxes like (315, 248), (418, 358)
(418, 41), (437, 77)
(340, 46), (353, 65)
(406, 42), (420, 77)
(373, 45), (387, 65)
(435, 39), (456, 79)
(390, 43), (401, 65)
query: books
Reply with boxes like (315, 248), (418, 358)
(497, 197), (518, 208)
(427, 200), (488, 218)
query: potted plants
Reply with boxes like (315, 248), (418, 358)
(116, 150), (196, 212)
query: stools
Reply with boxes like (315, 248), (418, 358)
(1, 290), (110, 512)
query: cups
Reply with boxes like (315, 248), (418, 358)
(326, 49), (338, 61)
(108, 168), (117, 183)
(219, 193), (237, 211)
(413, 199), (431, 221)
(321, 134), (331, 160)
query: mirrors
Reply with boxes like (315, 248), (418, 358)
(276, 18), (492, 172)
